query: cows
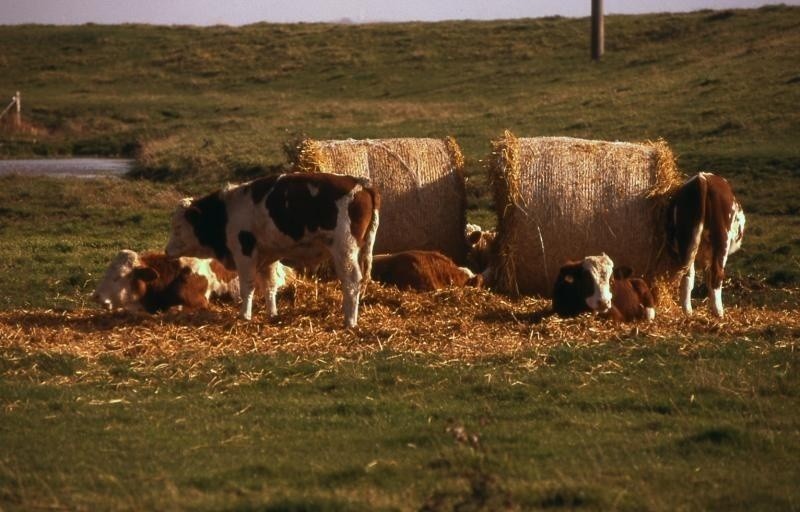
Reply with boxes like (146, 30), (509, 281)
(461, 220), (501, 287)
(88, 247), (294, 312)
(665, 169), (748, 324)
(550, 249), (660, 327)
(163, 170), (383, 331)
(370, 248), (473, 294)
